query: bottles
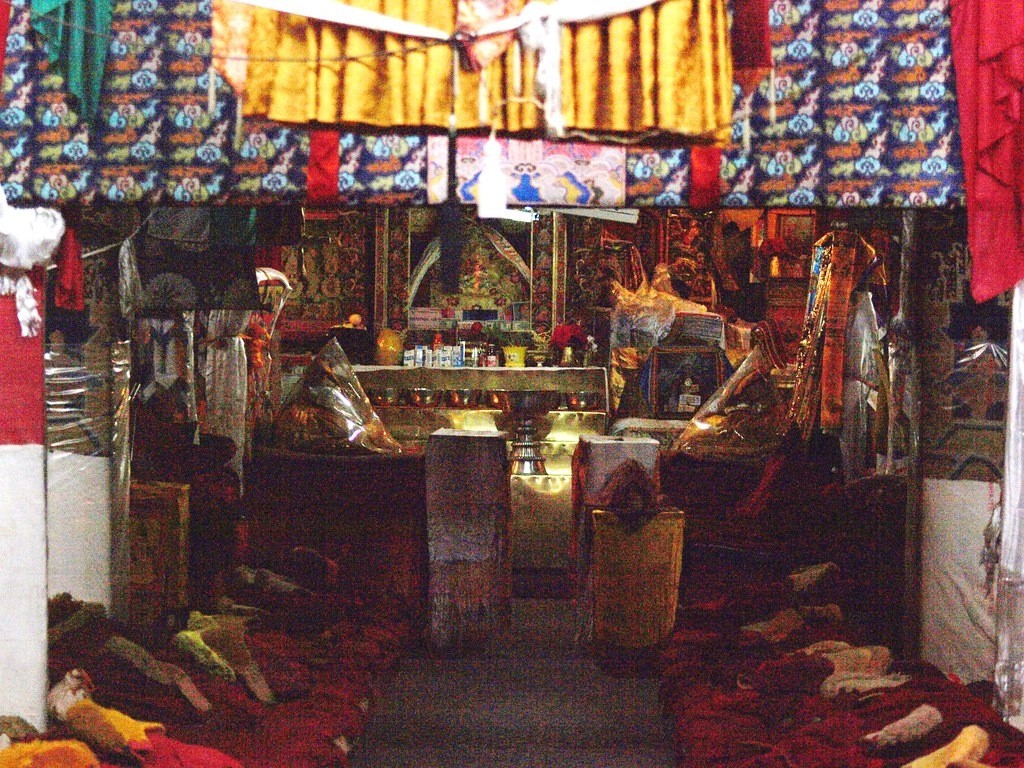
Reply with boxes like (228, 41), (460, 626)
(487, 344), (496, 366)
(433, 334), (443, 350)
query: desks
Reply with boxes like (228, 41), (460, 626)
(350, 367), (611, 570)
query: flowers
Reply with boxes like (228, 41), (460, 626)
(548, 322), (591, 352)
(471, 322), (483, 334)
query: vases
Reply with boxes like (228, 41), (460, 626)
(558, 346), (577, 367)
